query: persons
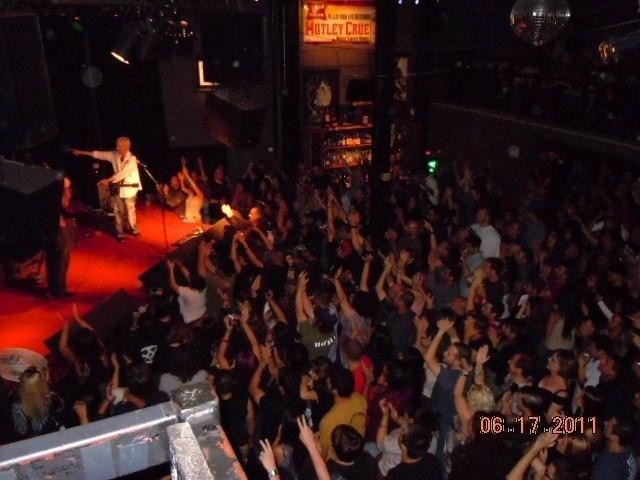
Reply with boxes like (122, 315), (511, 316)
(0, 16), (639, 480)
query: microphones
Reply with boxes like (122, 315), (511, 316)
(136, 159), (148, 168)
